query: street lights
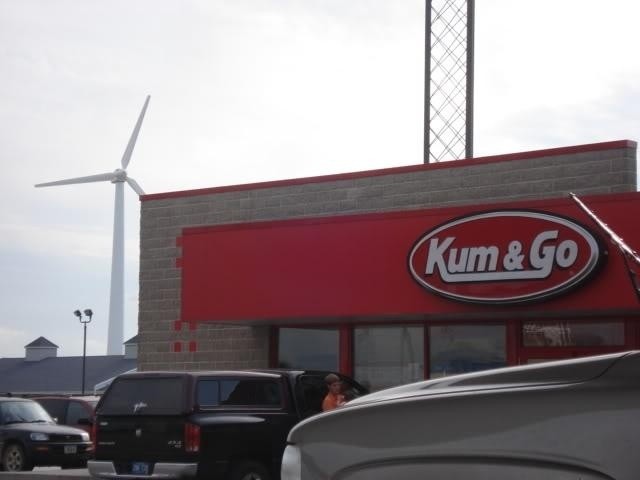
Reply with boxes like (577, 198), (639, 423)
(73, 308), (93, 396)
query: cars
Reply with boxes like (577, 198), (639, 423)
(27, 394), (103, 460)
(279, 346), (640, 480)
(0, 397), (94, 472)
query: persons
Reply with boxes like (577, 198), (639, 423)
(322, 371), (346, 414)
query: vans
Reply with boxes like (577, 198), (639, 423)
(87, 370), (369, 480)
